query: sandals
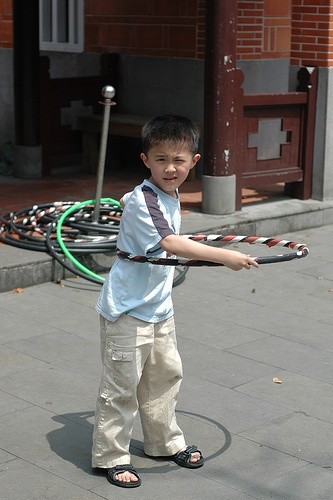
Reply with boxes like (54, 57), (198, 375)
(105, 465), (142, 488)
(174, 445), (204, 468)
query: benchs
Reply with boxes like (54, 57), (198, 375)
(77, 111), (205, 182)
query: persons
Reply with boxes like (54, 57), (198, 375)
(89, 113), (259, 488)
